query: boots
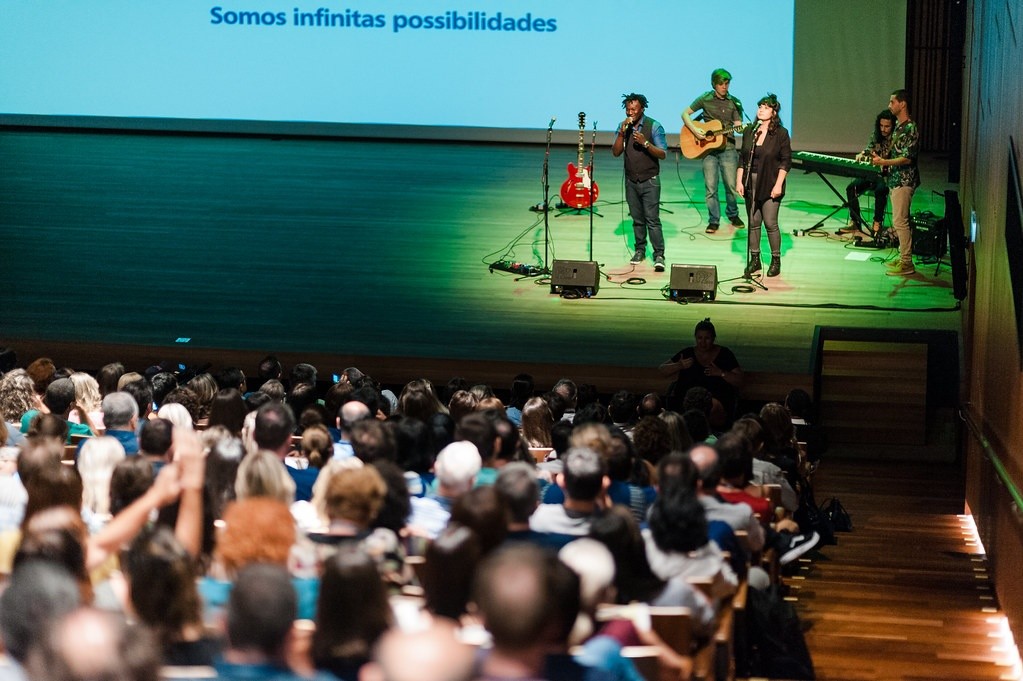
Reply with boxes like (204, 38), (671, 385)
(767, 255), (780, 276)
(744, 253), (762, 273)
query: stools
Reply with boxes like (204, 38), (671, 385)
(844, 189), (885, 226)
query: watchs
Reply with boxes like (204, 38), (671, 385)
(643, 140), (650, 149)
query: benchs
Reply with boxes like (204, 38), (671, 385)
(0, 411), (819, 681)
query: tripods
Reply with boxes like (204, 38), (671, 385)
(514, 121), (609, 282)
(718, 131), (768, 290)
(914, 211), (948, 276)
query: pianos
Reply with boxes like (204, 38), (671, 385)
(789, 150), (885, 238)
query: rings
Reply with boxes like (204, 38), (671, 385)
(634, 136), (636, 138)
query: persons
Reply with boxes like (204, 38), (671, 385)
(0, 348), (818, 681)
(839, 109), (897, 239)
(681, 68), (746, 232)
(872, 89), (922, 275)
(736, 94), (792, 276)
(612, 93), (667, 270)
(659, 320), (748, 404)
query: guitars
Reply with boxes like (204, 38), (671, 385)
(679, 119), (751, 161)
(560, 110), (599, 211)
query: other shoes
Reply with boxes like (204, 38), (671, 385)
(780, 531), (821, 565)
(840, 220), (860, 232)
(872, 219), (883, 236)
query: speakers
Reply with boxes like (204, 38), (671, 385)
(668, 264), (717, 300)
(550, 259), (600, 296)
(909, 211), (948, 259)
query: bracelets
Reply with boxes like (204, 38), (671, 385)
(720, 370), (726, 378)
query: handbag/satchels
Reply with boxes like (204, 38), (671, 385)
(820, 497), (852, 532)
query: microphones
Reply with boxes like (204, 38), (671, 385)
(548, 117), (556, 126)
(752, 120), (763, 133)
(625, 117), (633, 129)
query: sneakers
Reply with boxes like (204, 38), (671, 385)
(706, 223), (719, 232)
(729, 216), (744, 228)
(654, 256), (665, 269)
(886, 259), (900, 268)
(886, 261), (914, 275)
(630, 251), (645, 265)
